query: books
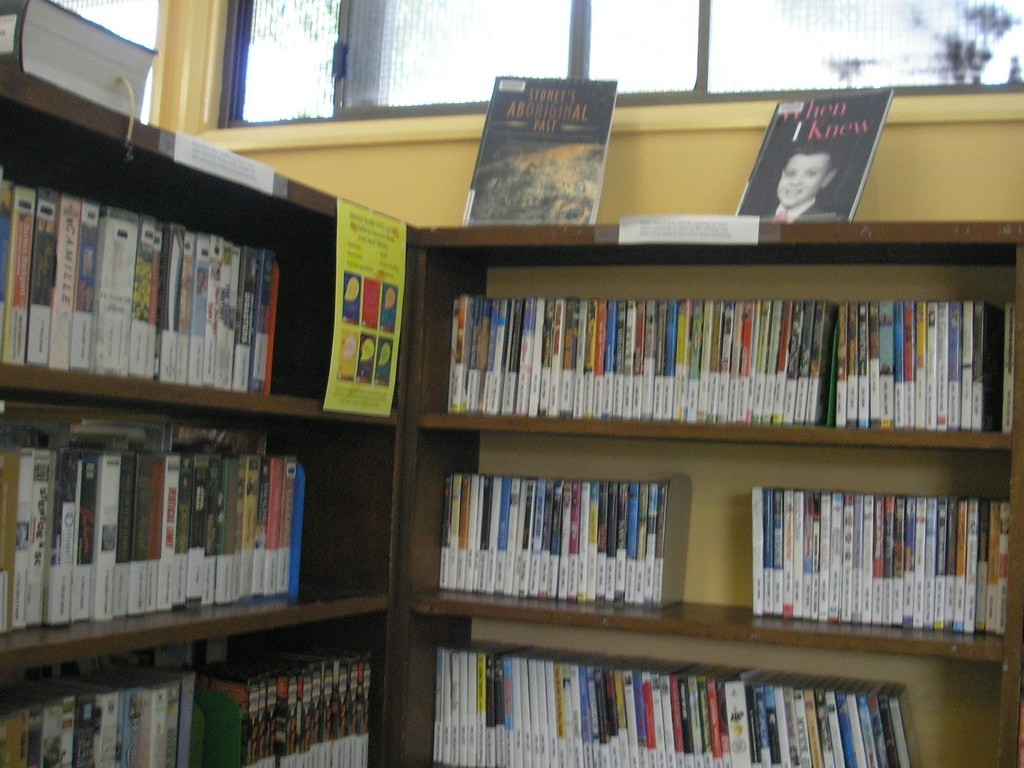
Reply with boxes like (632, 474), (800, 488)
(0, 0), (1018, 767)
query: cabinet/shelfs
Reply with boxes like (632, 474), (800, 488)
(0, 60), (417, 768)
(383, 216), (1024, 768)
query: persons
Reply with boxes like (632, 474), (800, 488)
(764, 136), (848, 221)
